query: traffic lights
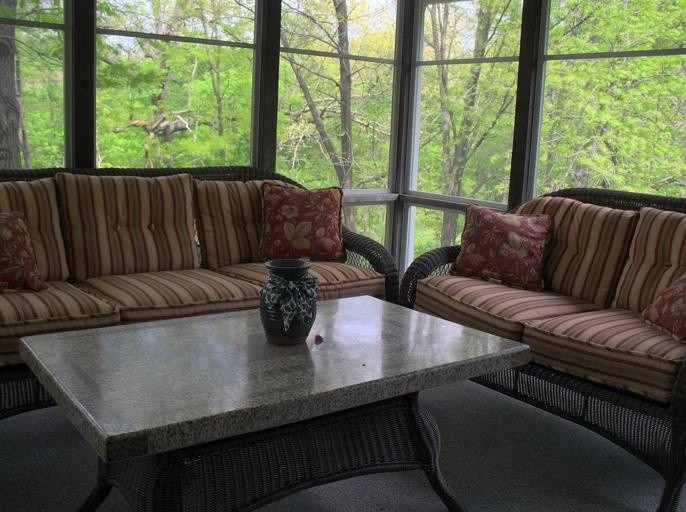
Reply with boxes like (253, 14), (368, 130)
(450, 202), (686, 345)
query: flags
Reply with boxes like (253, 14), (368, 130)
(258, 258), (316, 345)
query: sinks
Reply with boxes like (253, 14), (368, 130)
(0, 166), (400, 422)
(400, 188), (686, 512)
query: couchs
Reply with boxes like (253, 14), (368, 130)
(0, 166), (400, 422)
(400, 188), (686, 512)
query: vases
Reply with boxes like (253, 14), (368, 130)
(258, 258), (316, 345)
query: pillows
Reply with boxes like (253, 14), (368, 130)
(0, 172), (300, 281)
(450, 202), (686, 345)
(257, 180), (343, 265)
(1, 213), (48, 291)
